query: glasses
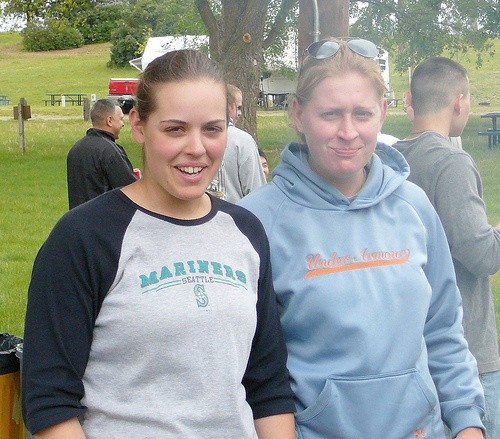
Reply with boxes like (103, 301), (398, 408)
(300, 39), (380, 75)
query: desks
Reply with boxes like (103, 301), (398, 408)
(0, 96), (8, 105)
(481, 112), (500, 145)
(47, 93), (87, 106)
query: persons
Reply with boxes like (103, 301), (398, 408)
(22, 49), (297, 438)
(205, 84), (268, 204)
(67, 98), (137, 210)
(233, 38), (487, 439)
(389, 56), (500, 439)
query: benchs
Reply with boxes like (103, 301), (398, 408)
(385, 98), (401, 107)
(0, 99), (10, 105)
(477, 130), (500, 149)
(41, 99), (73, 107)
(54, 100), (84, 106)
(487, 128), (500, 143)
